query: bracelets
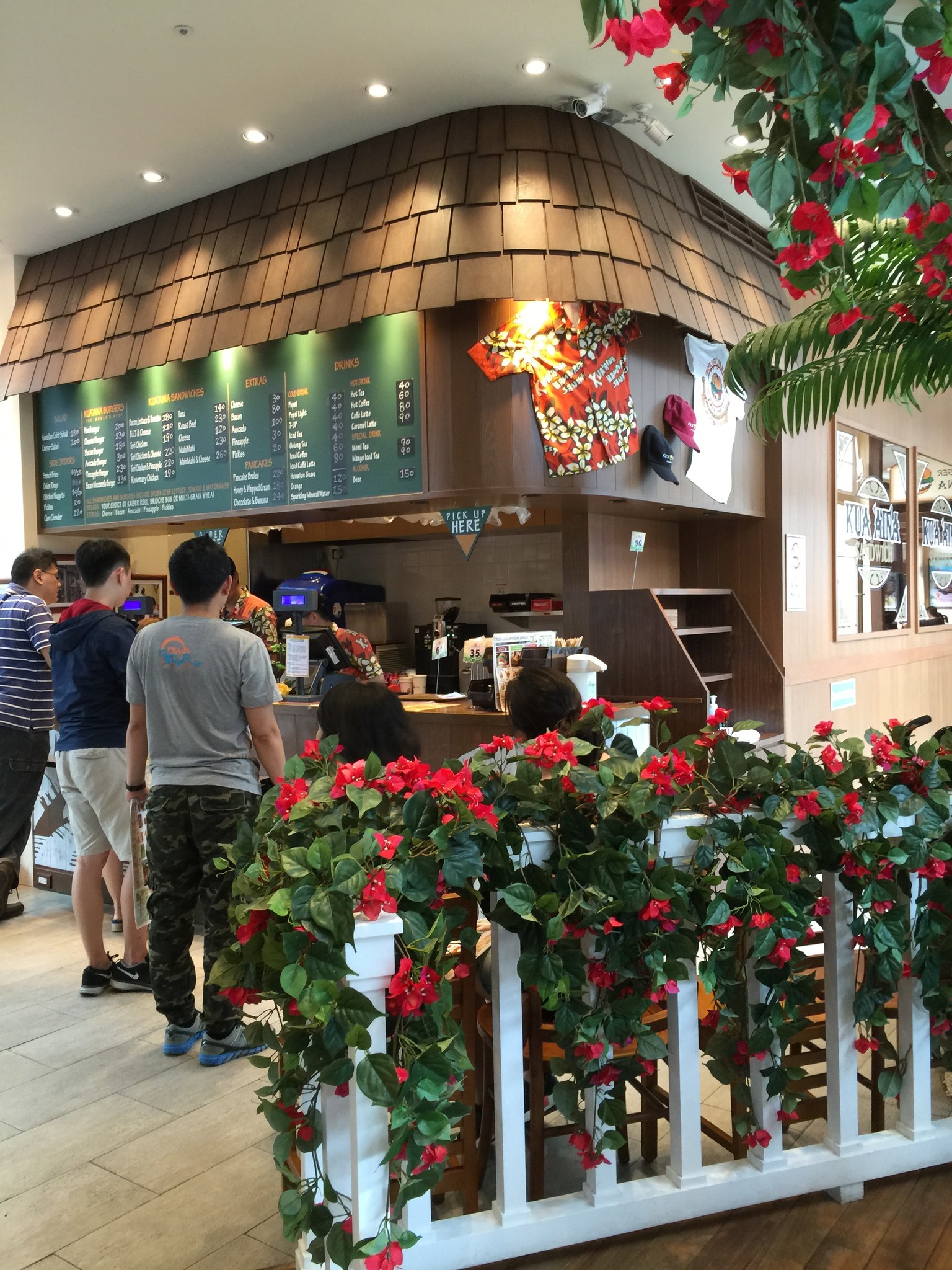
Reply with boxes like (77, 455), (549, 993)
(125, 780), (146, 792)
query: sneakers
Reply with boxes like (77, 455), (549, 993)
(80, 951), (119, 994)
(198, 1019), (275, 1065)
(163, 1009), (206, 1054)
(109, 957), (153, 990)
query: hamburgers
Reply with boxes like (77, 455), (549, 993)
(498, 653), (507, 664)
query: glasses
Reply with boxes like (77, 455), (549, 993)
(31, 569), (61, 583)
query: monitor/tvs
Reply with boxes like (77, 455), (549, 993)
(279, 625), (352, 673)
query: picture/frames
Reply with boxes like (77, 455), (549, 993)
(112, 574), (168, 620)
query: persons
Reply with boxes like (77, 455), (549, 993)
(0, 548), (61, 922)
(123, 534), (287, 1067)
(301, 602), (385, 685)
(315, 680), (421, 768)
(505, 667), (582, 741)
(100, 617), (165, 932)
(48, 538), (150, 998)
(220, 558), (278, 664)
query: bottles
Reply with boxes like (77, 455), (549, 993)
(392, 671), (399, 685)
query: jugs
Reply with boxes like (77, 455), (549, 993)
(566, 654), (608, 702)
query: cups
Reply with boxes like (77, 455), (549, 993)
(399, 677), (412, 695)
(412, 675), (427, 694)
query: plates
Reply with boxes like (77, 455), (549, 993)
(720, 727), (760, 744)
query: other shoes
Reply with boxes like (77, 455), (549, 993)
(0, 901), (24, 920)
(0, 860), (19, 909)
(111, 916), (124, 933)
